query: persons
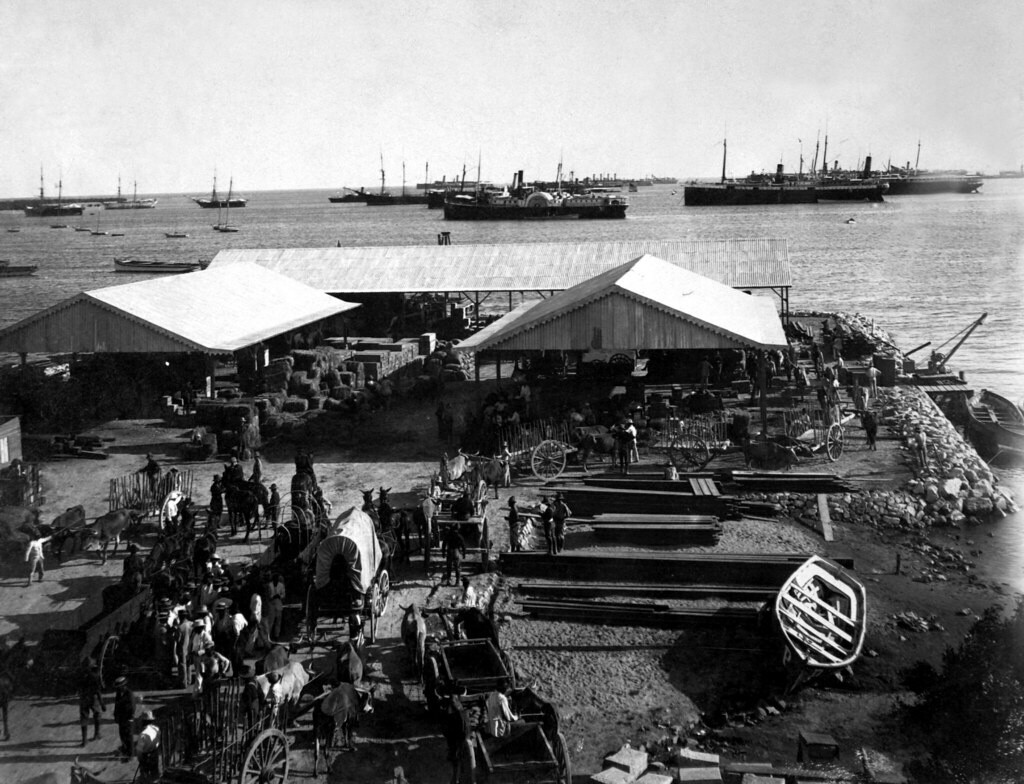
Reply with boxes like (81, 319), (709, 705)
(296, 447), (322, 491)
(542, 492), (572, 556)
(506, 493), (523, 554)
(119, 448), (288, 692)
(113, 676), (137, 764)
(133, 708), (163, 784)
(69, 656), (107, 748)
(482, 679), (526, 738)
(470, 341), (881, 488)
(23, 528), (53, 586)
(437, 521), (467, 585)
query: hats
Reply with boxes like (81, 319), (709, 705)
(238, 416), (247, 423)
(541, 497), (550, 504)
(507, 496), (516, 503)
(220, 558), (231, 567)
(138, 710), (156, 724)
(213, 597), (233, 610)
(229, 457), (239, 462)
(125, 543), (141, 553)
(160, 598), (172, 606)
(113, 676), (129, 690)
(555, 493), (565, 499)
(209, 553), (220, 561)
(253, 451), (261, 458)
(202, 640), (215, 650)
(269, 484), (277, 489)
(197, 605), (209, 612)
(211, 474), (221, 482)
(80, 656), (96, 669)
(265, 672), (282, 684)
(154, 612), (170, 620)
(435, 475), (443, 482)
(452, 521), (461, 529)
(240, 665), (256, 677)
(176, 611), (190, 617)
(169, 493), (177, 500)
(501, 441), (508, 447)
(190, 619), (208, 629)
(212, 579), (224, 586)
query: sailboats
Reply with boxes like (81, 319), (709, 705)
(49, 198), (68, 229)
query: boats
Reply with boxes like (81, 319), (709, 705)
(75, 228), (90, 231)
(329, 163), (628, 221)
(774, 555), (868, 686)
(91, 211), (105, 235)
(111, 257), (210, 274)
(105, 189), (155, 209)
(213, 203), (238, 233)
(23, 189), (84, 218)
(191, 189), (246, 209)
(1, 260), (39, 278)
(8, 229), (20, 233)
(685, 140), (982, 206)
(958, 386), (1024, 456)
(163, 232), (186, 238)
(112, 234), (124, 236)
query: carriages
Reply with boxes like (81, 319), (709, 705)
(83, 414), (577, 784)
(573, 404), (849, 478)
